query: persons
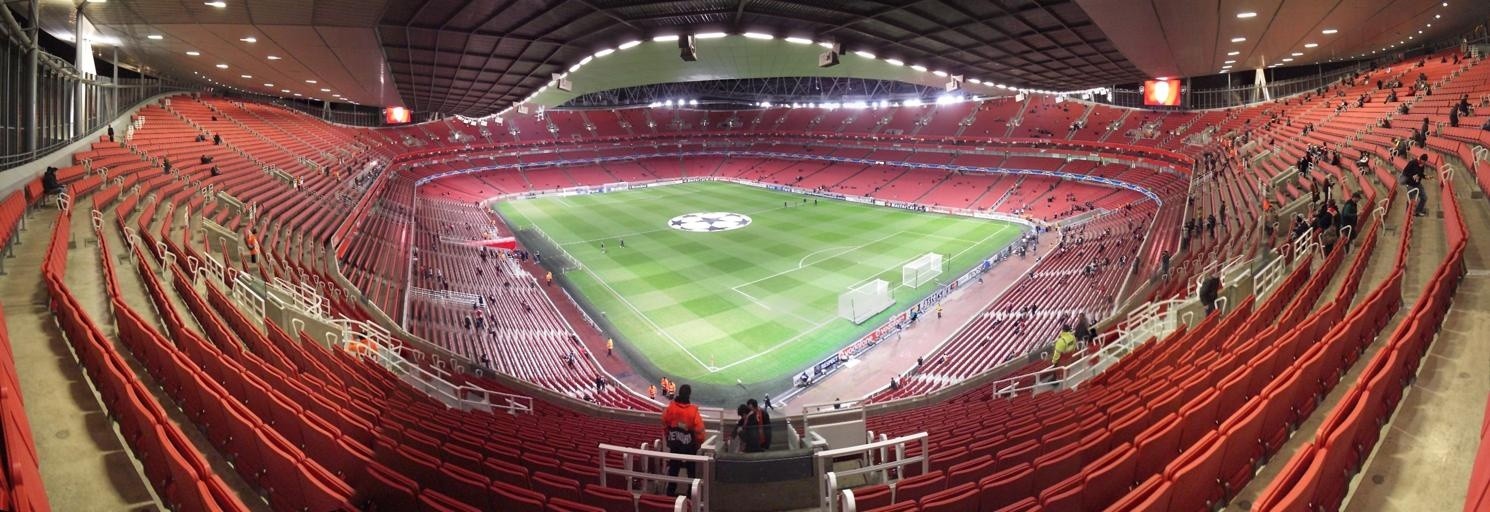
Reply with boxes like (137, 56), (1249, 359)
(661, 382), (705, 498)
(42, 45), (1487, 411)
(734, 404), (762, 451)
(731, 398), (773, 450)
